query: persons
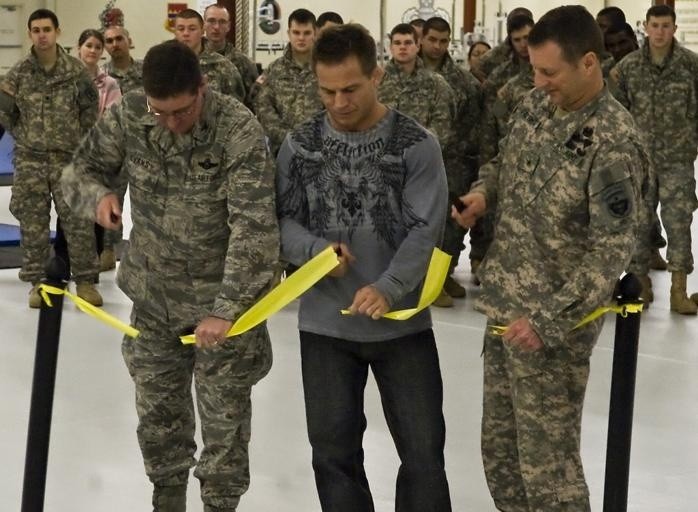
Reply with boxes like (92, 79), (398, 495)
(453, 7), (650, 509)
(60, 40), (280, 512)
(275, 23), (452, 512)
(0, 0), (698, 314)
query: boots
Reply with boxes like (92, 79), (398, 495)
(670, 271), (698, 315)
(638, 274), (653, 310)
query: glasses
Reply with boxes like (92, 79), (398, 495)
(146, 89), (200, 120)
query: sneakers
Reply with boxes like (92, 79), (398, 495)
(28, 281), (46, 308)
(650, 247), (667, 270)
(420, 273), (466, 307)
(100, 248), (116, 272)
(77, 278), (103, 306)
